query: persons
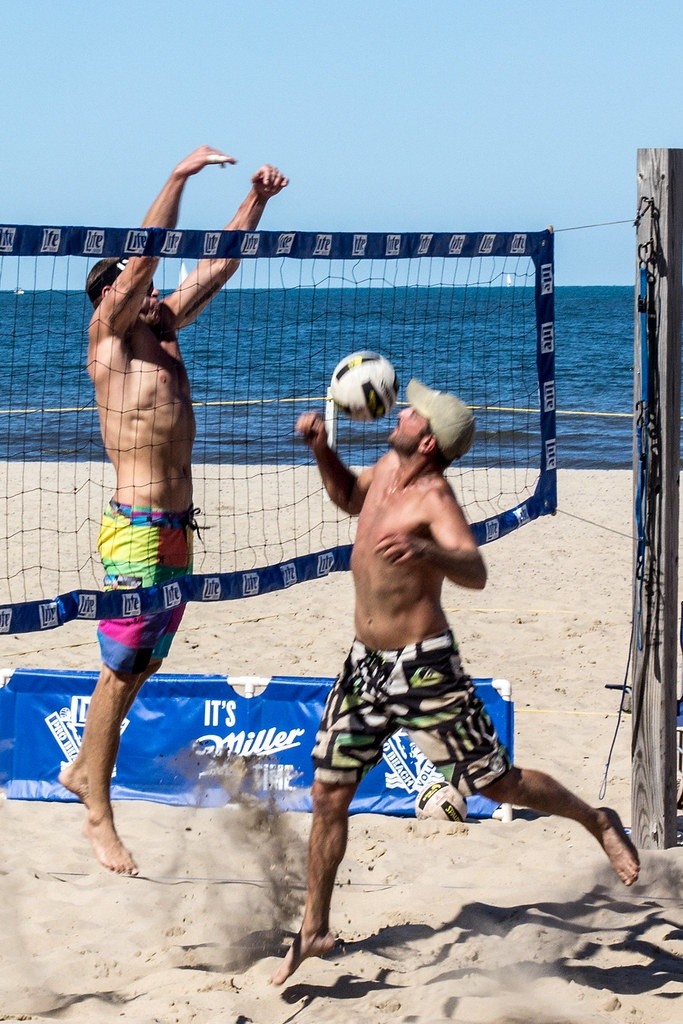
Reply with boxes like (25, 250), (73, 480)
(59, 145), (291, 877)
(266, 378), (641, 986)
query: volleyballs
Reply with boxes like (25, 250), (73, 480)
(329, 349), (400, 423)
(413, 780), (469, 823)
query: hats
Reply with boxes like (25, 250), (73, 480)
(405, 378), (478, 461)
